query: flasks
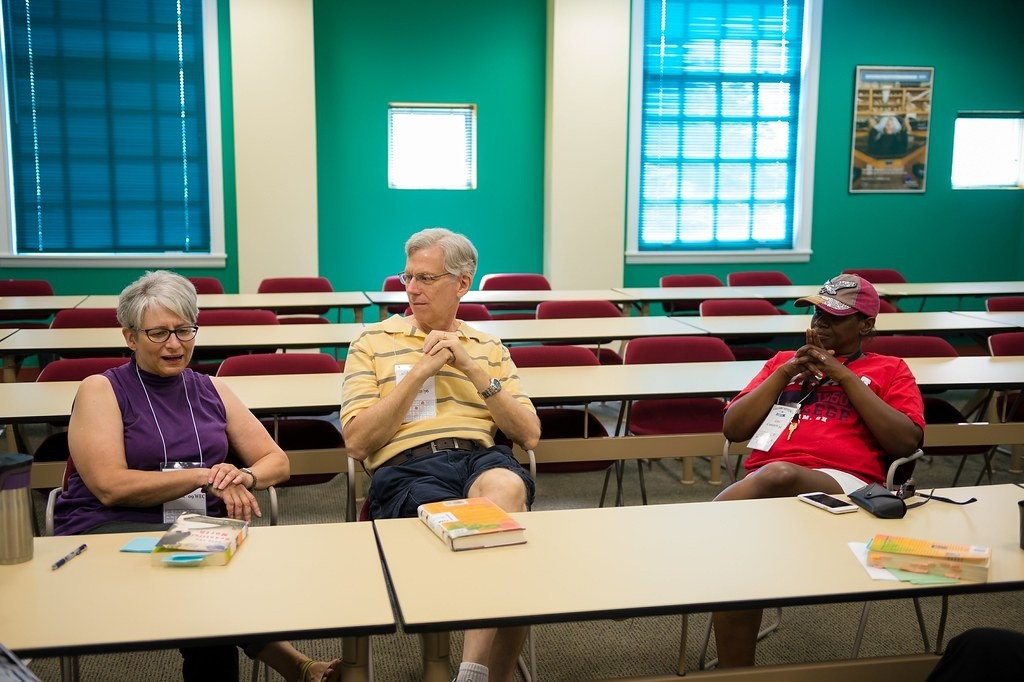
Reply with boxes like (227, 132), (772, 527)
(0, 453), (35, 565)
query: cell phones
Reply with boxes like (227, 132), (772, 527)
(799, 492), (860, 514)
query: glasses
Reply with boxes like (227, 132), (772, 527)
(398, 271), (451, 285)
(896, 477), (977, 510)
(133, 326), (198, 343)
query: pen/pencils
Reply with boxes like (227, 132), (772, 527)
(52, 544), (87, 571)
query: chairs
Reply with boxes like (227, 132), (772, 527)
(0, 269), (1024, 682)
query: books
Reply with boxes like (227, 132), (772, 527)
(150, 511), (248, 567)
(867, 533), (992, 584)
(417, 497), (528, 552)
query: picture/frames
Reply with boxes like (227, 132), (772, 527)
(849, 65), (934, 194)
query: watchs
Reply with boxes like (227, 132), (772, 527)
(476, 378), (502, 400)
(240, 466), (257, 493)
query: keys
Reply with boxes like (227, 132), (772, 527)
(786, 423), (798, 440)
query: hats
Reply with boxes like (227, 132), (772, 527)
(794, 273), (880, 317)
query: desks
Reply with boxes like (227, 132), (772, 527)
(362, 316), (708, 344)
(870, 281), (1024, 311)
(0, 323), (365, 382)
(951, 311), (1024, 332)
(668, 311), (1020, 351)
(517, 359), (769, 510)
(372, 484), (1024, 682)
(0, 521), (395, 682)
(612, 286), (823, 315)
(0, 328), (18, 340)
(0, 295), (87, 313)
(0, 373), (344, 425)
(901, 356), (1024, 390)
(75, 292), (371, 323)
(364, 290), (635, 323)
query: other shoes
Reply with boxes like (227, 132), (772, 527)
(297, 659), (339, 682)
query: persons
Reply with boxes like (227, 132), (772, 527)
(56, 269), (342, 682)
(340, 227), (542, 682)
(711, 273), (926, 670)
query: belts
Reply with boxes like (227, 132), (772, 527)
(378, 437), (487, 467)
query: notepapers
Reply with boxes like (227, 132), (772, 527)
(120, 537), (161, 553)
